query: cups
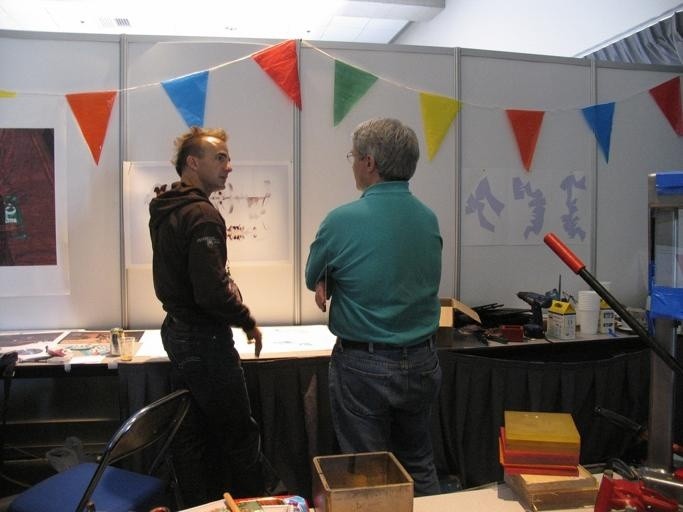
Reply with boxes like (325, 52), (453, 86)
(118, 336), (135, 361)
(577, 290), (600, 336)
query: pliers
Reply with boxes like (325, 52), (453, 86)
(473, 330), (507, 346)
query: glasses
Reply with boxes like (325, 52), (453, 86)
(346, 151), (361, 164)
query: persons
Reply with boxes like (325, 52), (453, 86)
(149, 126), (289, 499)
(302, 116), (445, 498)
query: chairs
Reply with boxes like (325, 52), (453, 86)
(8, 388), (192, 512)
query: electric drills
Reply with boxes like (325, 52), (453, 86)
(511, 292), (553, 339)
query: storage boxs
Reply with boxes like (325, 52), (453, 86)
(436, 297), (481, 347)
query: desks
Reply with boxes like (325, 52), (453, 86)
(0, 323), (639, 506)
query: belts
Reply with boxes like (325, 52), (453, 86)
(341, 335), (437, 349)
